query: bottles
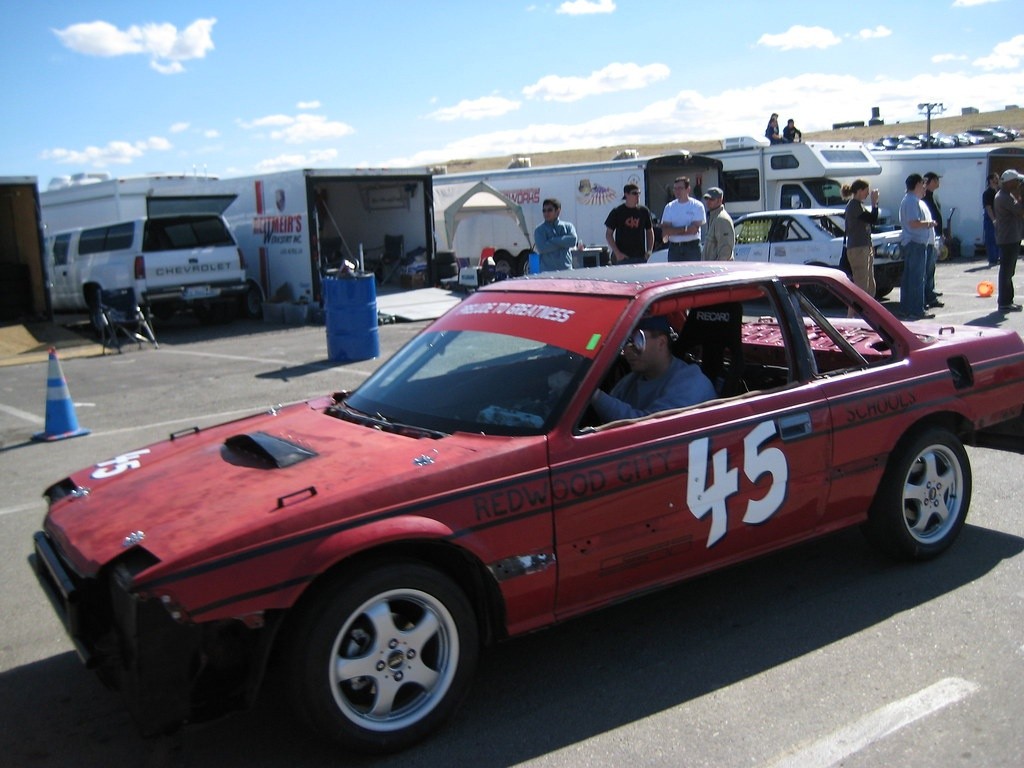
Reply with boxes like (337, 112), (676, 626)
(578, 240), (583, 251)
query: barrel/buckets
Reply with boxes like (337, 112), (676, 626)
(323, 273), (379, 363)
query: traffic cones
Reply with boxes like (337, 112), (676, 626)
(32, 348), (92, 443)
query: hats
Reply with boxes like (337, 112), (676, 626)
(1001, 170), (1024, 182)
(623, 184), (638, 199)
(703, 187), (723, 199)
(633, 315), (666, 332)
(924, 173), (942, 180)
(906, 174), (928, 185)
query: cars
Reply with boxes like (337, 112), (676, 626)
(643, 206), (957, 310)
(865, 125), (1022, 153)
(28, 259), (1024, 743)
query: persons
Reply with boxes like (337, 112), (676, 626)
(702, 186), (735, 262)
(545, 315), (717, 424)
(993, 168), (1024, 313)
(533, 198), (578, 274)
(898, 172), (945, 320)
(841, 179), (880, 318)
(982, 172), (1000, 268)
(765, 113), (801, 146)
(604, 184), (655, 265)
(658, 176), (707, 262)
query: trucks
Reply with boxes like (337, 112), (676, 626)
(692, 135), (894, 241)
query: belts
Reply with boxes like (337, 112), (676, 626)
(671, 242), (687, 246)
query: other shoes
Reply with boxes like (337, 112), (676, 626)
(909, 311), (935, 319)
(998, 303), (1023, 311)
(929, 300), (944, 307)
(936, 293), (943, 296)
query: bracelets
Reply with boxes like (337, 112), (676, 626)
(685, 226), (687, 232)
(992, 220), (997, 224)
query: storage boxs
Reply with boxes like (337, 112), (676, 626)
(400, 274), (424, 290)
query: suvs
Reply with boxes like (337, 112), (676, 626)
(41, 192), (247, 336)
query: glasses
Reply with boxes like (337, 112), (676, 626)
(630, 192), (641, 195)
(543, 208), (552, 212)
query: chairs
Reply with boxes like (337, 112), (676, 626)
(81, 280), (159, 355)
(373, 234), (404, 285)
(768, 224), (790, 241)
(676, 301), (749, 400)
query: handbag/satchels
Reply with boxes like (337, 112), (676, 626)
(839, 246), (853, 277)
